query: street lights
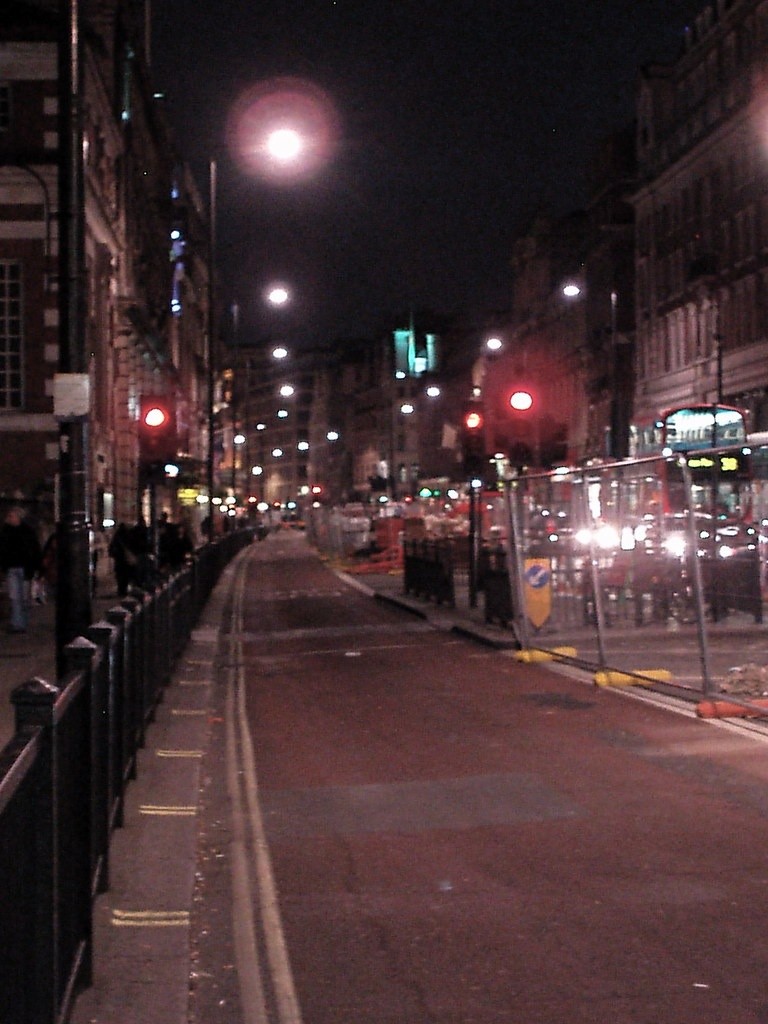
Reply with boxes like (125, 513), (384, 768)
(205, 115), (311, 547)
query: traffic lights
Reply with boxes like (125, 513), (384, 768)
(138, 392), (179, 480)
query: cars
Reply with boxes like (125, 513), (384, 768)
(439, 485), (768, 623)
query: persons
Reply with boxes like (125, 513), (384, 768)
(472, 531), (507, 629)
(0, 498), (42, 635)
(108, 511), (197, 597)
